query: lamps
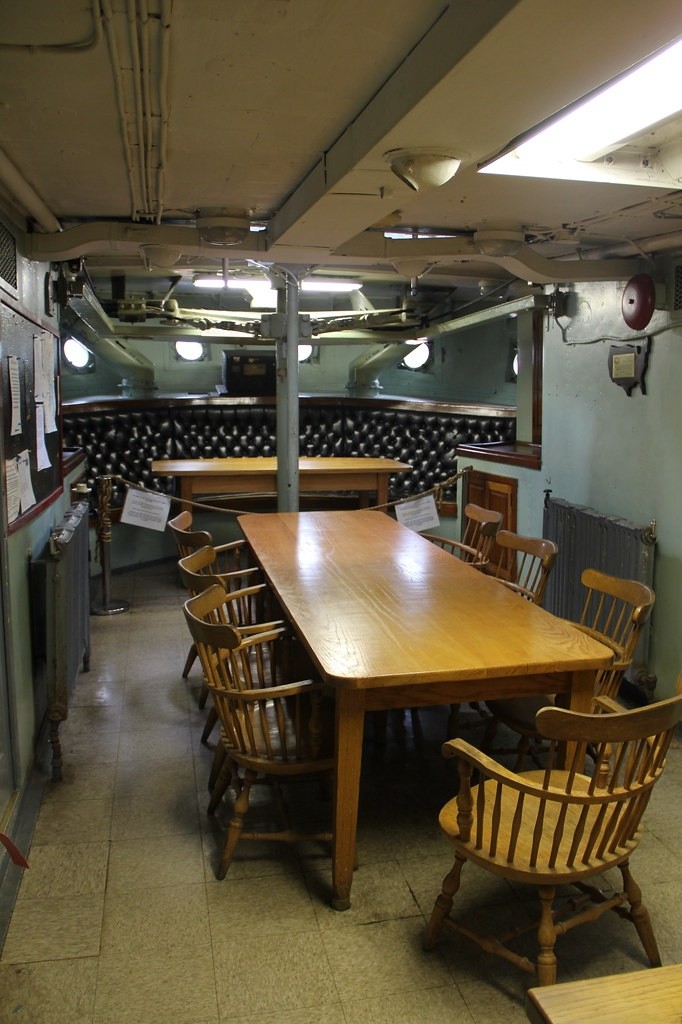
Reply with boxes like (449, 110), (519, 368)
(191, 275), (364, 292)
(552, 230), (580, 245)
(197, 208), (250, 246)
(390, 257), (429, 276)
(373, 213), (402, 227)
(140, 244), (183, 267)
(383, 147), (471, 188)
(477, 281), (508, 297)
(471, 230), (525, 256)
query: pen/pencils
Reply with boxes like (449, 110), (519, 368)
(188, 392), (209, 394)
(529, 444), (541, 447)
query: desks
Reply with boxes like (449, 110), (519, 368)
(525, 965), (682, 1024)
(236, 510), (613, 912)
(150, 455), (415, 589)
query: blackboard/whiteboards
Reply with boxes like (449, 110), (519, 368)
(1, 289), (63, 536)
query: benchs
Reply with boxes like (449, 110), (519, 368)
(58, 396), (517, 528)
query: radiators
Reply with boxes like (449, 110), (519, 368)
(32, 484), (92, 722)
(541, 489), (660, 692)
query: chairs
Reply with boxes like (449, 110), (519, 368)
(411, 501), (656, 782)
(423, 673), (682, 988)
(168, 511), (360, 880)
